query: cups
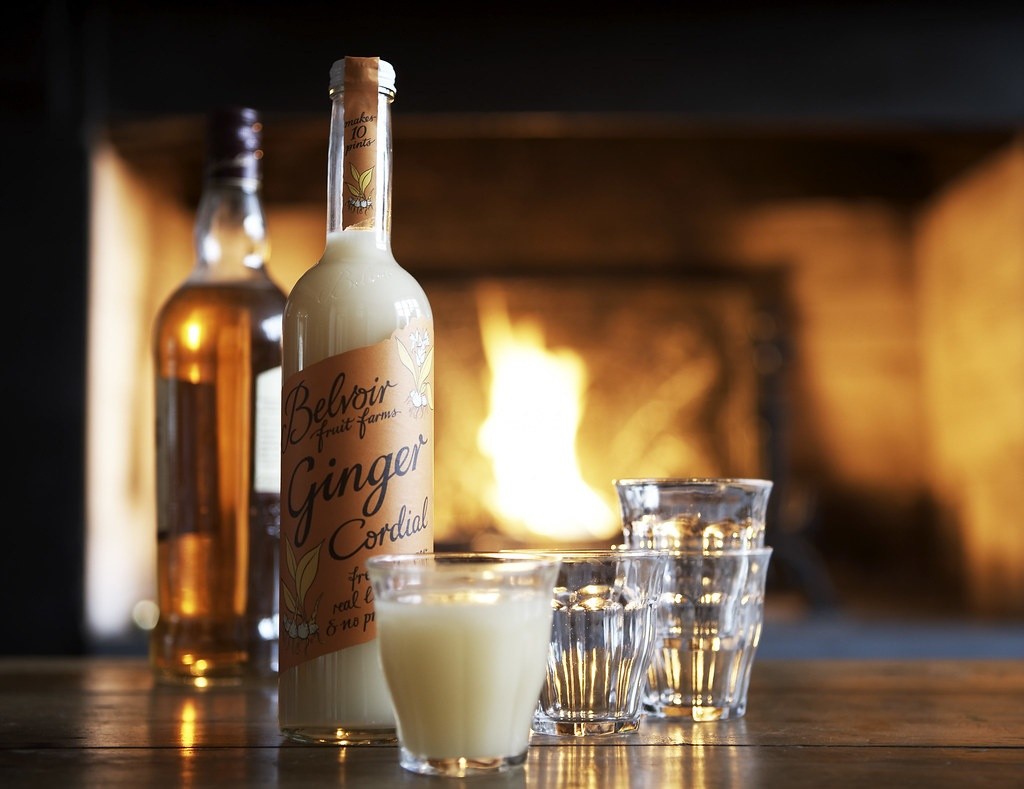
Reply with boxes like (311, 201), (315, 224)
(499, 549), (669, 736)
(613, 548), (775, 722)
(365, 550), (563, 778)
(613, 479), (773, 550)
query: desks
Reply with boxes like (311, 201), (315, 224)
(0, 659), (1024, 789)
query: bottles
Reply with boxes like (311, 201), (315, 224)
(280, 52), (436, 749)
(152, 113), (288, 691)
